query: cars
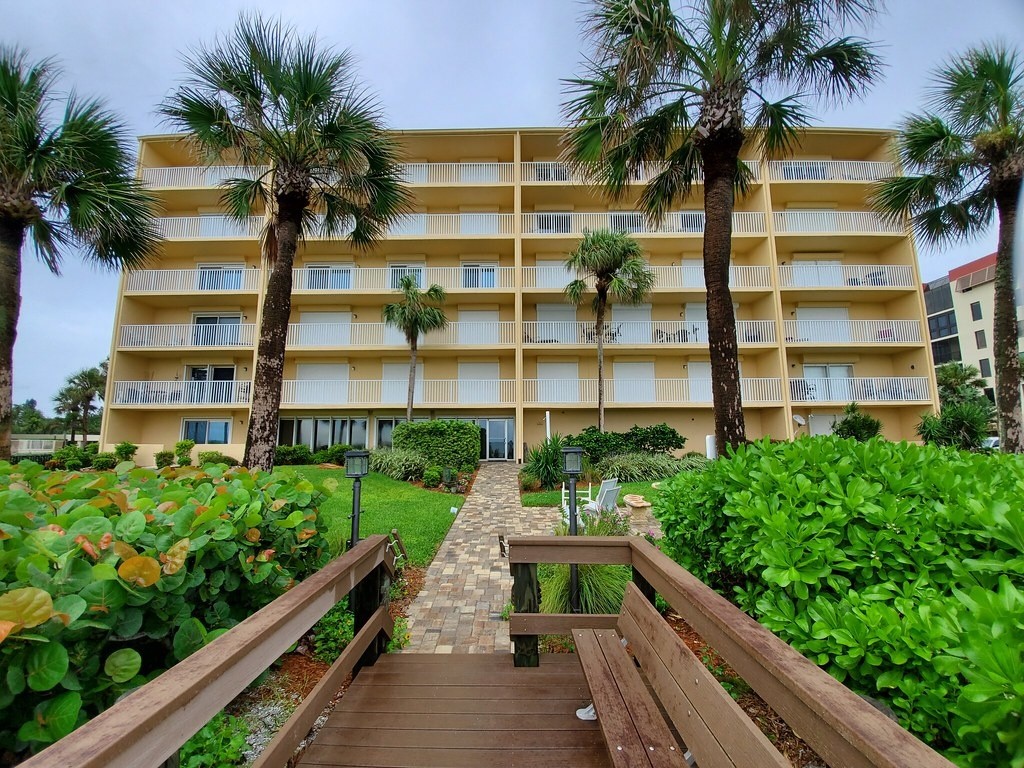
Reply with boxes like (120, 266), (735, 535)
(970, 436), (1000, 453)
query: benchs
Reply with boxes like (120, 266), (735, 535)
(623, 493), (651, 524)
(571, 582), (790, 768)
(791, 379), (816, 399)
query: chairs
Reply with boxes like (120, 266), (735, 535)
(656, 328), (670, 342)
(577, 478), (624, 526)
(581, 322), (622, 343)
(674, 329), (690, 342)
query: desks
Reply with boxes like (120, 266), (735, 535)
(147, 390), (167, 403)
(651, 482), (664, 496)
(594, 324), (610, 343)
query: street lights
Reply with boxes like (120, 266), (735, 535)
(561, 444), (585, 614)
(345, 449), (371, 610)
